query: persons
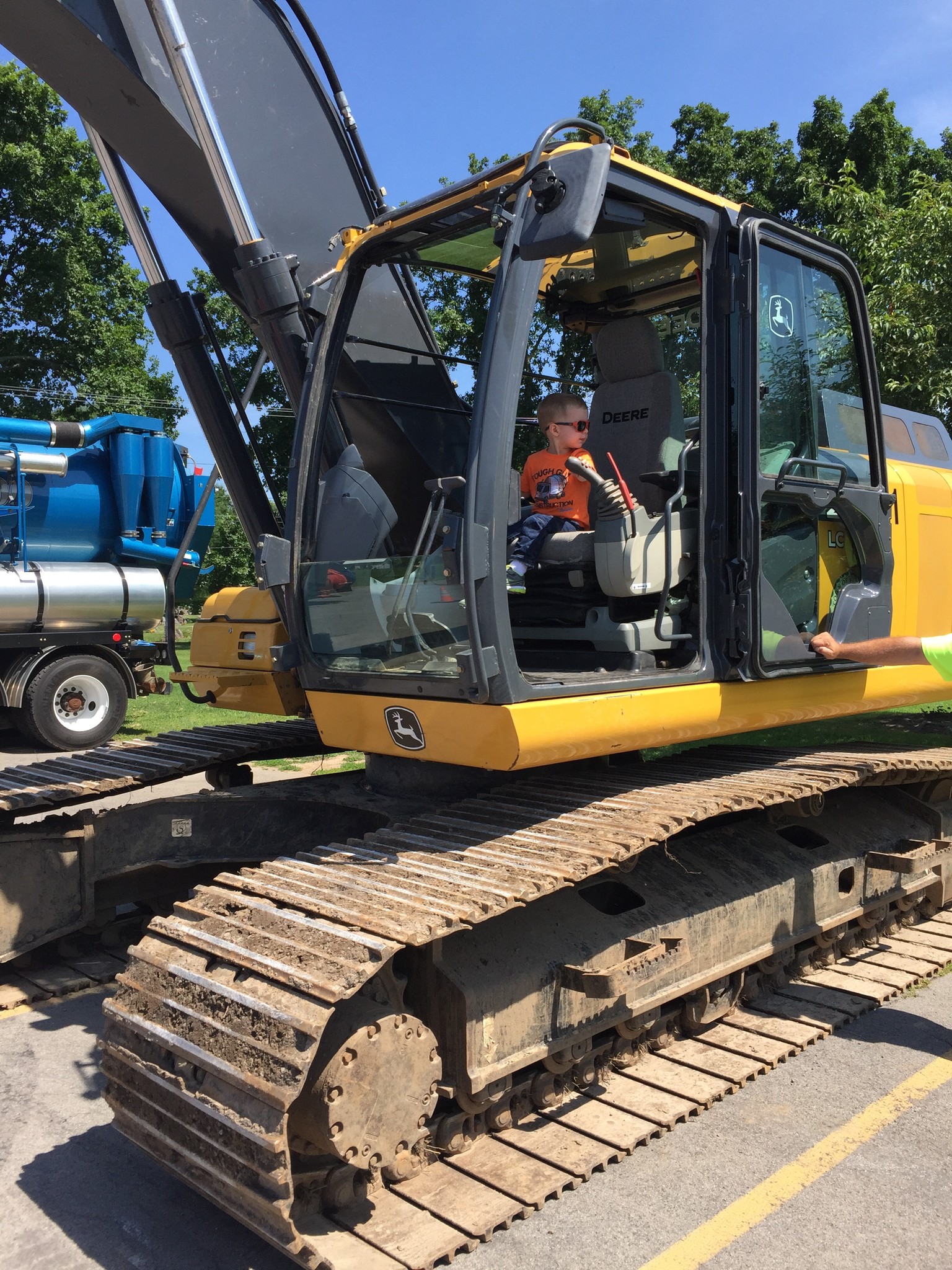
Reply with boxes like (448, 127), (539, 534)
(811, 633), (952, 680)
(762, 628), (815, 661)
(503, 392), (598, 595)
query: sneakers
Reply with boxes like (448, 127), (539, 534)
(506, 565), (526, 595)
(459, 598), (466, 609)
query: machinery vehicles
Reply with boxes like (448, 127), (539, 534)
(1, 409), (219, 754)
(0, 4), (952, 1270)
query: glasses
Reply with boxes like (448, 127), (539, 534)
(544, 420), (590, 433)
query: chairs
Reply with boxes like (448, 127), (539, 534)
(537, 315), (685, 562)
